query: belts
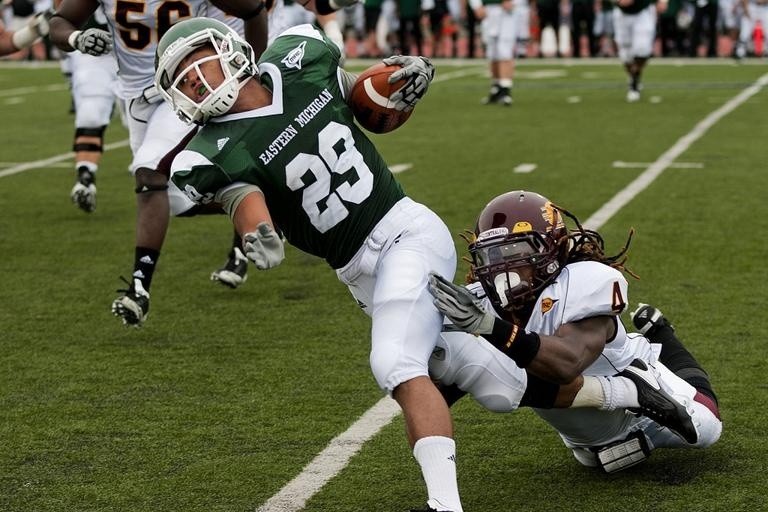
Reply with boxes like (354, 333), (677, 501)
(336, 200), (414, 282)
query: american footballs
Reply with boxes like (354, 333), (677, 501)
(347, 62), (415, 134)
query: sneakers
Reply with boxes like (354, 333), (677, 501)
(71, 173), (97, 213)
(110, 277), (149, 324)
(629, 302), (665, 335)
(217, 245), (250, 288)
(626, 81), (641, 103)
(483, 85), (513, 106)
(612, 357), (700, 446)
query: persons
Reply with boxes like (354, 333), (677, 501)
(1, 1), (76, 114)
(153, 15), (723, 512)
(465, 1), (534, 108)
(608, 1), (670, 105)
(48, 1), (249, 328)
(366, 0), (488, 60)
(207, 2), (366, 67)
(427, 188), (723, 473)
(515, 2), (619, 59)
(655, 1), (768, 59)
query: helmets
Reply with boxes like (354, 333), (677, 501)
(466, 190), (570, 311)
(153, 15), (260, 127)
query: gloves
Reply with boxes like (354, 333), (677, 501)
(427, 270), (497, 335)
(243, 221), (284, 270)
(74, 27), (114, 56)
(27, 8), (55, 36)
(383, 53), (435, 113)
